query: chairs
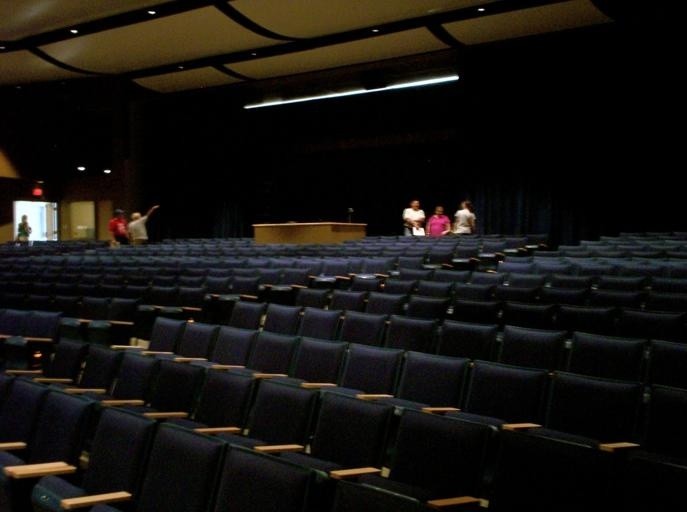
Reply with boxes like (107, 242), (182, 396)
(0, 237), (687, 511)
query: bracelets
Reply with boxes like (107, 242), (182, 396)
(426, 233), (431, 234)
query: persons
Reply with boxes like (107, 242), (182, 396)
(425, 206), (451, 237)
(127, 204), (160, 245)
(109, 208), (129, 245)
(402, 200), (426, 236)
(18, 215), (32, 242)
(452, 199), (476, 235)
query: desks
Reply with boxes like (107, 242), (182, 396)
(250, 223), (369, 244)
